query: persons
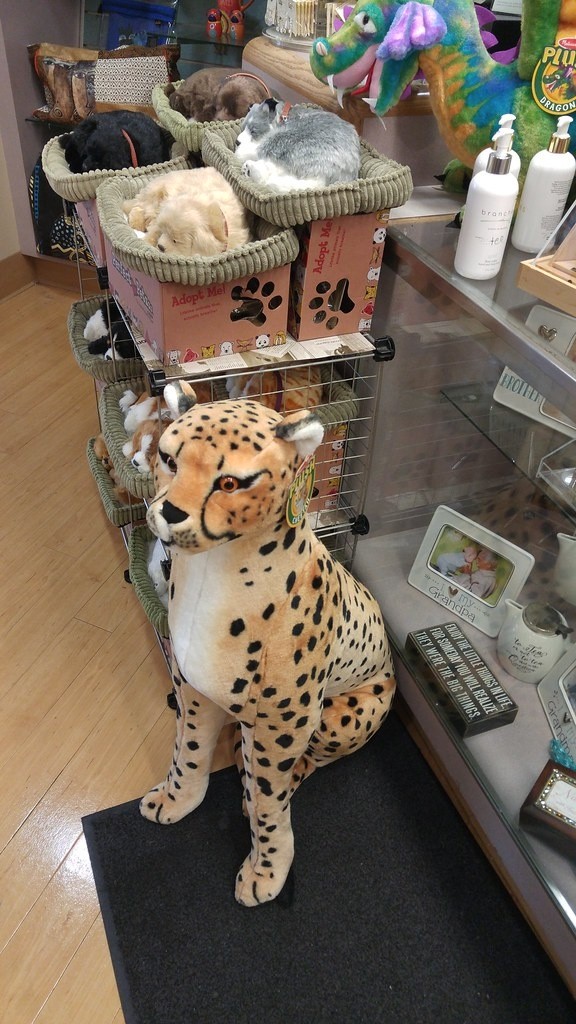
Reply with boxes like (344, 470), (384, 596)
(430, 543), (498, 601)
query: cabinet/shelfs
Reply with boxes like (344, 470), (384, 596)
(361, 212), (576, 1000)
(27, 118), (104, 293)
(83, 0), (269, 81)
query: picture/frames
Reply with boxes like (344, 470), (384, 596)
(536, 641), (576, 766)
(407, 505), (535, 639)
(493, 305), (576, 434)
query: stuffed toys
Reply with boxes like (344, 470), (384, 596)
(122, 165), (252, 258)
(94, 434), (146, 505)
(84, 297), (142, 362)
(163, 67), (271, 124)
(58, 110), (177, 174)
(309, 0), (576, 230)
(234, 97), (362, 192)
(118, 371), (218, 473)
(226, 360), (322, 418)
(140, 380), (397, 906)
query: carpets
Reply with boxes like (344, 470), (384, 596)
(82, 710), (576, 1024)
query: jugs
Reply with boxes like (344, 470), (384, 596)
(496, 598), (574, 684)
(553, 534), (576, 605)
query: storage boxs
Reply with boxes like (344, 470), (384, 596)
(74, 198), (390, 367)
(304, 421), (346, 514)
